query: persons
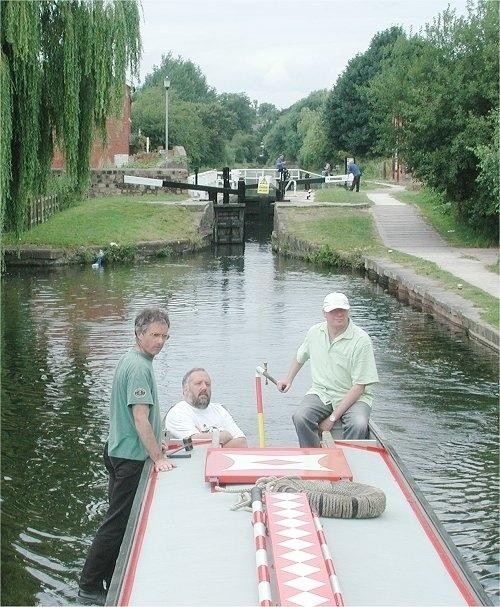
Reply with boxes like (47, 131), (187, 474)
(348, 160), (362, 192)
(322, 161), (331, 175)
(76, 307), (173, 606)
(275, 153), (288, 174)
(277, 288), (378, 448)
(304, 172), (312, 200)
(161, 367), (249, 449)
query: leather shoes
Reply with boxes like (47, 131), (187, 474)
(76, 587), (108, 606)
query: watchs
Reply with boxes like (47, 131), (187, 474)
(328, 414), (337, 422)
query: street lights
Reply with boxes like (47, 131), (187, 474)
(164, 75), (171, 151)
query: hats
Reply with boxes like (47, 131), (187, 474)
(323, 293), (350, 312)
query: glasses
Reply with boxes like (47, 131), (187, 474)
(147, 333), (170, 340)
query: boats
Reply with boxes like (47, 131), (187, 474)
(102, 361), (499, 607)
